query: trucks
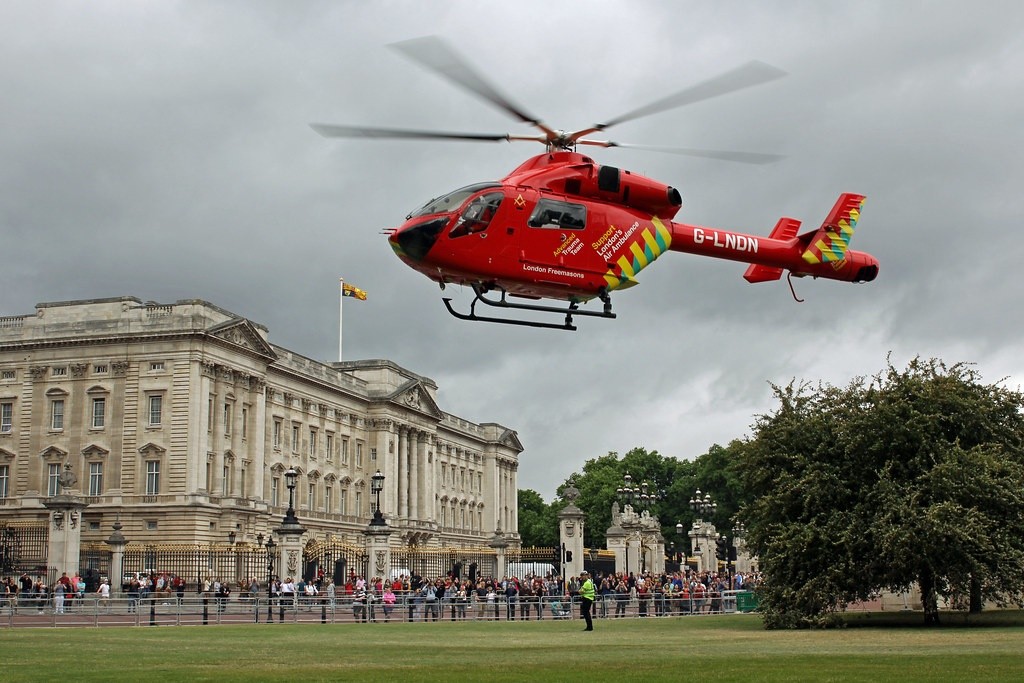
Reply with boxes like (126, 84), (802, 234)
(507, 562), (559, 580)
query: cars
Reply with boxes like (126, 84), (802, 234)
(149, 573), (186, 591)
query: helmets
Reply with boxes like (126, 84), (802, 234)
(580, 571), (588, 575)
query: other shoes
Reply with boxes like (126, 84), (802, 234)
(584, 628), (593, 631)
(128, 611), (131, 612)
(133, 611), (135, 612)
(39, 611), (45, 615)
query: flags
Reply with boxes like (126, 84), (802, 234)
(342, 283), (367, 300)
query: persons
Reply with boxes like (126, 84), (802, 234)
(0, 572), (86, 614)
(202, 576), (336, 611)
(345, 573), (579, 623)
(579, 571), (595, 631)
(127, 572), (185, 613)
(97, 579), (110, 606)
(589, 568), (767, 618)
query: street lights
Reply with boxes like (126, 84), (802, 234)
(624, 530), (639, 577)
(265, 535), (277, 623)
(640, 533), (656, 574)
(587, 542), (598, 619)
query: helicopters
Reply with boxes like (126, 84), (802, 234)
(308, 34), (880, 331)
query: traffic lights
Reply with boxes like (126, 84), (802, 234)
(730, 546), (737, 560)
(676, 552), (682, 563)
(715, 538), (728, 561)
(566, 551), (572, 562)
(553, 545), (561, 562)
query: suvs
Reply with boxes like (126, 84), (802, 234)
(122, 571), (153, 586)
